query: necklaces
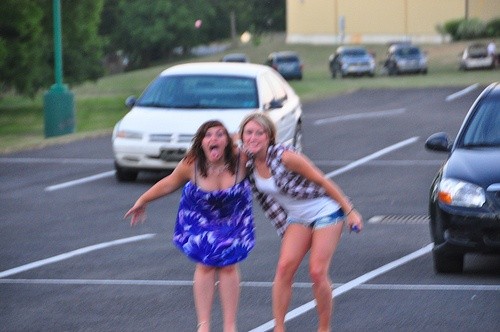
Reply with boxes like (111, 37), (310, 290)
(205, 163), (228, 175)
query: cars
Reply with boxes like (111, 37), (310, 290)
(425, 82), (500, 274)
(330, 46), (377, 77)
(264, 51), (304, 81)
(384, 43), (429, 76)
(460, 44), (495, 69)
(112, 61), (303, 182)
(222, 54), (249, 62)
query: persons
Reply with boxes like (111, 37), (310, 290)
(183, 113), (362, 332)
(124, 120), (257, 332)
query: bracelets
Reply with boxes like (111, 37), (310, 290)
(346, 206), (354, 216)
